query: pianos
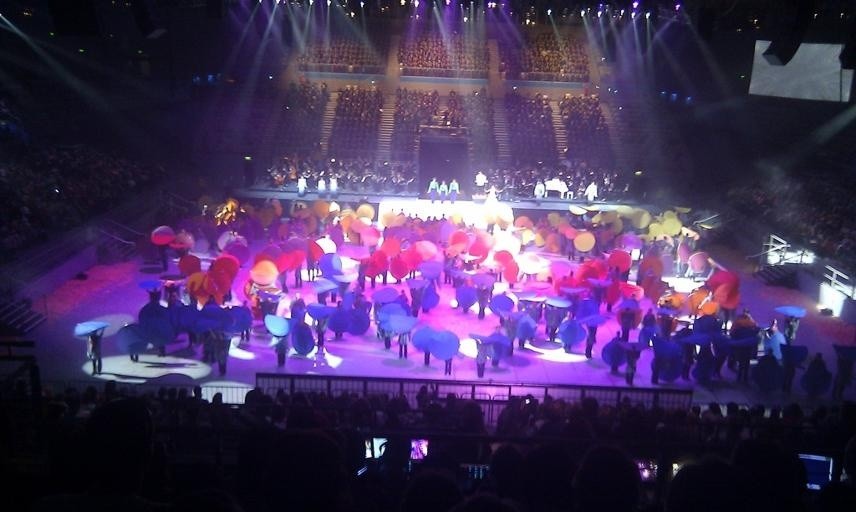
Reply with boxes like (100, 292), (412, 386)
(545, 180), (569, 199)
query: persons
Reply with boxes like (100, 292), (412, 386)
(1, 20), (856, 512)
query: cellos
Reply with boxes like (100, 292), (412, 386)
(287, 158), (297, 181)
